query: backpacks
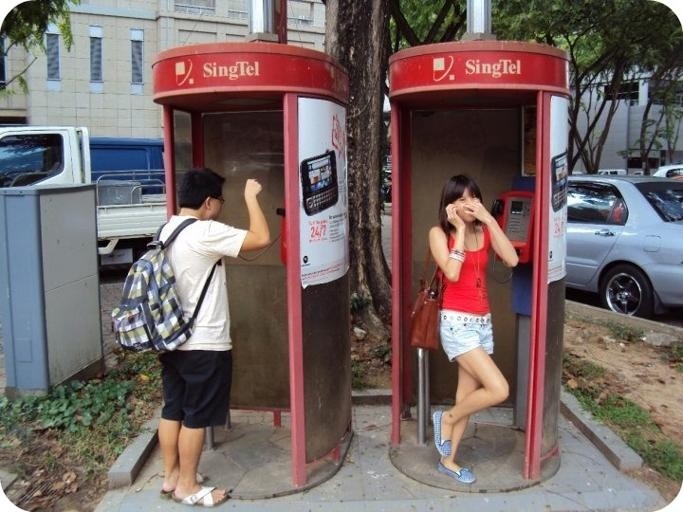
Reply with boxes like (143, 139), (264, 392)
(111, 218), (223, 353)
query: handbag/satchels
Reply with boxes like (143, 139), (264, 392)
(406, 225), (451, 349)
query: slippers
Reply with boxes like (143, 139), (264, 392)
(159, 472), (209, 501)
(172, 485), (229, 508)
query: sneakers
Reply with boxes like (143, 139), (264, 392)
(437, 457), (476, 483)
(433, 410), (452, 457)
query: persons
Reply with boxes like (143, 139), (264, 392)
(150, 165), (272, 509)
(426, 169), (519, 487)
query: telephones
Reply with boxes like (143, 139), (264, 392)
(490, 191), (535, 264)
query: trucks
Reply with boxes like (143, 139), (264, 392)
(0, 127), (168, 270)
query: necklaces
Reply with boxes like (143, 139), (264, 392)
(461, 223), (482, 288)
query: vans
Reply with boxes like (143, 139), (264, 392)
(79, 137), (166, 194)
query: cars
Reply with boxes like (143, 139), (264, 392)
(565, 165), (683, 318)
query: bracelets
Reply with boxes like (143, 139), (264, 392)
(448, 248), (467, 264)
